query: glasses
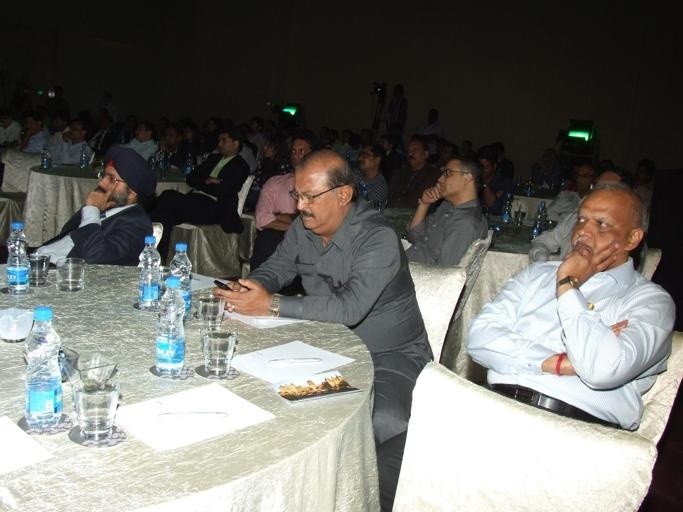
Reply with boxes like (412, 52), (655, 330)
(441, 168), (469, 178)
(286, 184), (346, 204)
(96, 170), (127, 186)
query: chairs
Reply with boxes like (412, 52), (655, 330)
(393, 360), (658, 511)
(1, 116), (683, 439)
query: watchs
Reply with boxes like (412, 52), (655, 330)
(268, 292), (282, 318)
(556, 275), (580, 289)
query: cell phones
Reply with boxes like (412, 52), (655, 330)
(214, 280), (233, 291)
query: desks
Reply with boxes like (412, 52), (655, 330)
(3, 262), (381, 512)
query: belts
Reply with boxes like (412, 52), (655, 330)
(487, 384), (625, 430)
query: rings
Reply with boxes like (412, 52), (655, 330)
(231, 304), (235, 311)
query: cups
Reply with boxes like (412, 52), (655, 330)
(73, 381), (124, 449)
(55, 256), (87, 296)
(373, 199), (385, 216)
(200, 325), (237, 378)
(22, 345), (78, 386)
(63, 352), (119, 394)
(195, 293), (225, 332)
(25, 251), (53, 286)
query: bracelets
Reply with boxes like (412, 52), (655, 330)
(556, 352), (566, 376)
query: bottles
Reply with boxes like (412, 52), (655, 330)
(134, 232), (193, 381)
(148, 149), (194, 183)
(5, 222), (33, 294)
(483, 172), (567, 244)
(96, 159), (107, 179)
(358, 182), (370, 205)
(23, 305), (63, 433)
(79, 143), (88, 170)
(40, 148), (50, 170)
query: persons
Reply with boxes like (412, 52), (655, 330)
(465, 179), (676, 432)
(210, 147), (434, 512)
